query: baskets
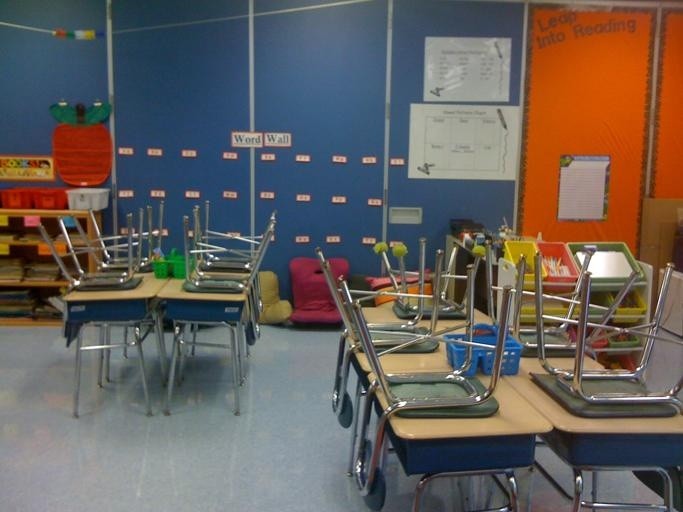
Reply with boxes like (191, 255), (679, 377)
(153, 248), (196, 279)
(444, 325), (524, 375)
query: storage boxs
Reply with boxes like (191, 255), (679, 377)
(0, 186), (111, 211)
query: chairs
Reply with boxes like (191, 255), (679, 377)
(36, 199), (278, 419)
(288, 255), (353, 326)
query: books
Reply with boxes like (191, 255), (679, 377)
(0, 233), (79, 322)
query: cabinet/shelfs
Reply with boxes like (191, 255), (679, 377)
(0, 209), (101, 326)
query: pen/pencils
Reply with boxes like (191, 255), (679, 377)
(543, 255), (564, 277)
(492, 216), (522, 248)
